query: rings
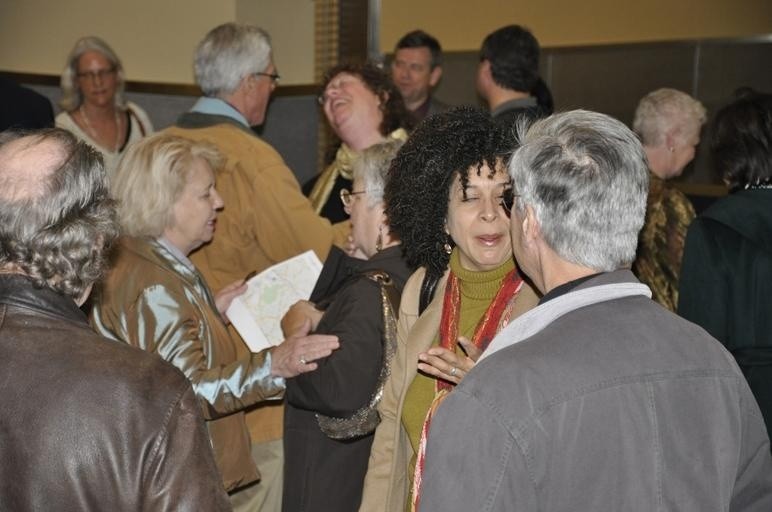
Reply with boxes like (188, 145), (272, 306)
(300, 354), (306, 364)
(450, 367), (457, 377)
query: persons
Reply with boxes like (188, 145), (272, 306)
(279, 134), (422, 511)
(301, 59), (409, 225)
(87, 129), (340, 512)
(165, 21), (356, 512)
(359, 106), (544, 512)
(55, 36), (155, 179)
(627, 87), (708, 313)
(389, 27), (455, 129)
(415, 106), (771, 512)
(0, 127), (235, 511)
(475, 24), (554, 138)
(676, 89), (772, 448)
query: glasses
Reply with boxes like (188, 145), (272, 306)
(76, 65), (116, 85)
(255, 73), (280, 85)
(502, 189), (520, 212)
(340, 188), (366, 206)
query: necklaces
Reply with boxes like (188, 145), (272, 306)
(78, 105), (121, 155)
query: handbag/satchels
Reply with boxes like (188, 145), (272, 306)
(314, 270), (398, 440)
